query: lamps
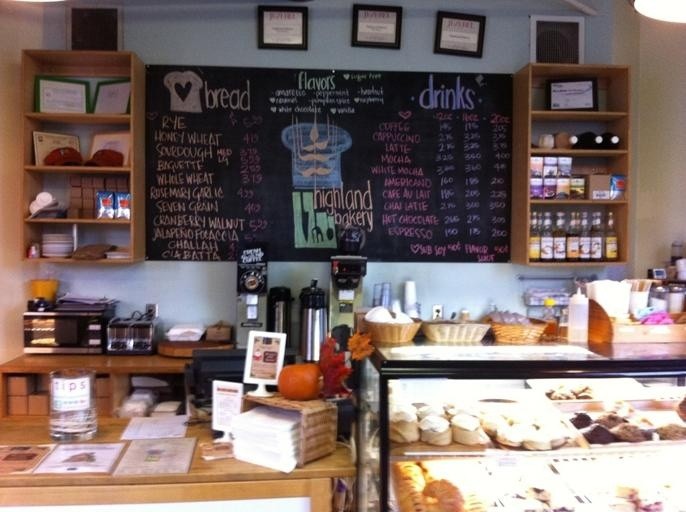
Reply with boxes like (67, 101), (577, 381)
(628, 0), (686, 23)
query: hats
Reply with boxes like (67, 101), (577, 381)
(44, 148), (83, 166)
(85, 149), (124, 167)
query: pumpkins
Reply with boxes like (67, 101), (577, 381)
(278, 356), (324, 400)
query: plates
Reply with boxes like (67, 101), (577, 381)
(40, 232), (72, 258)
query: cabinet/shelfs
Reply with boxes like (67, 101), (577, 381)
(510, 63), (630, 265)
(0, 349), (192, 416)
(20, 47), (146, 262)
(350, 332), (685, 511)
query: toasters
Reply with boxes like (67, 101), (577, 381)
(105, 314), (160, 355)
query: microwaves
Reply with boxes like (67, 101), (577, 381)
(21, 307), (117, 354)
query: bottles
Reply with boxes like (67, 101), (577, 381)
(668, 284), (685, 314)
(540, 300), (555, 339)
(671, 239), (685, 268)
(485, 302), (498, 315)
(266, 284), (296, 349)
(552, 130), (578, 148)
(567, 288), (588, 344)
(299, 277), (327, 362)
(556, 307), (569, 340)
(529, 210), (617, 262)
(571, 131), (602, 147)
(459, 309), (471, 319)
(650, 284), (669, 312)
(600, 133), (620, 148)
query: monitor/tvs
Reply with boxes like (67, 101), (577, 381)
(192, 349), (296, 404)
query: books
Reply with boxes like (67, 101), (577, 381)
(104, 247), (129, 259)
(52, 301), (117, 311)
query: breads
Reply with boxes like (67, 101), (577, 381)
(389, 382), (686, 450)
(390, 460), (486, 512)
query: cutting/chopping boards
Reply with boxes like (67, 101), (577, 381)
(154, 339), (235, 358)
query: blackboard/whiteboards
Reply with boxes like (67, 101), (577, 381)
(146, 64), (512, 261)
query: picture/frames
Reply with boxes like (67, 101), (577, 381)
(434, 9), (485, 58)
(257, 5), (307, 51)
(352, 2), (402, 50)
(35, 74), (89, 115)
(90, 79), (130, 114)
(67, 4), (123, 51)
(546, 77), (598, 112)
(87, 129), (130, 165)
(32, 130), (80, 166)
(528, 14), (585, 64)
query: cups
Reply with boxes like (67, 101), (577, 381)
(48, 368), (97, 443)
(628, 290), (650, 315)
(401, 281), (420, 320)
(30, 277), (60, 312)
(371, 281), (395, 309)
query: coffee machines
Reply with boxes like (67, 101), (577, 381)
(322, 253), (369, 344)
(233, 258), (267, 350)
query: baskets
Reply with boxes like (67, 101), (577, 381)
(484, 314), (550, 344)
(362, 317), (422, 347)
(422, 320), (492, 343)
(241, 394), (340, 467)
